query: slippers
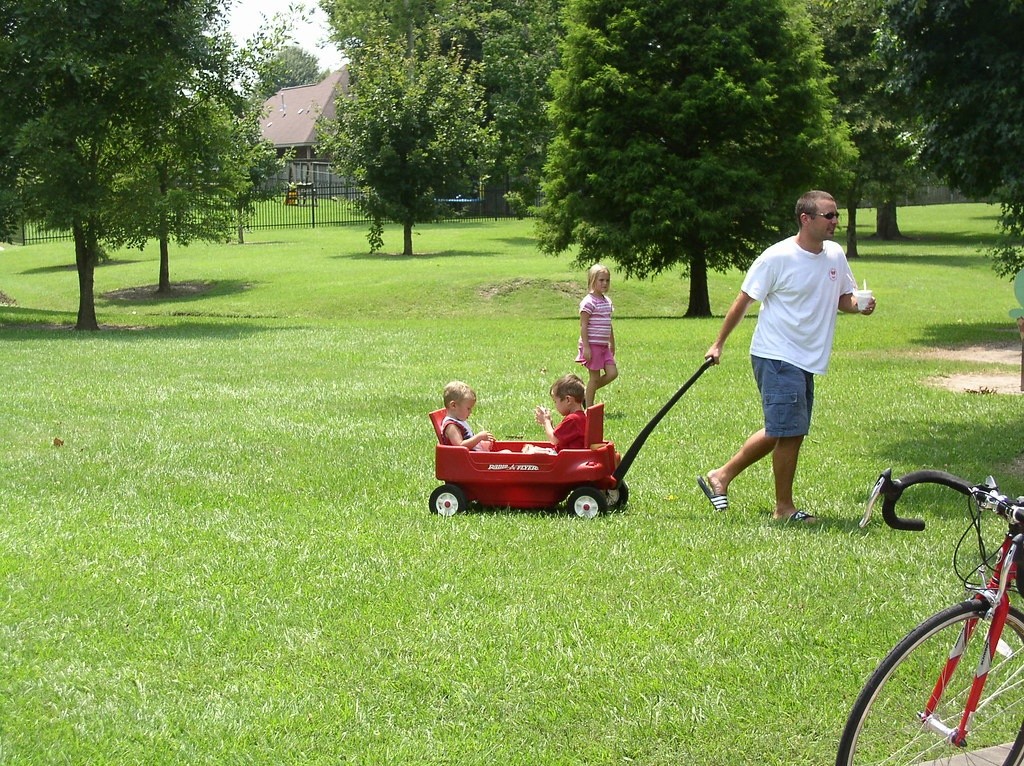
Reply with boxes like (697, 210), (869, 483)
(697, 475), (727, 511)
(770, 509), (818, 526)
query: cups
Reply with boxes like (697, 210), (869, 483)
(853, 290), (873, 311)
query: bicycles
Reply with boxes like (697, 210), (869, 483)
(836, 463), (1024, 766)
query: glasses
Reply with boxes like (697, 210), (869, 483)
(805, 211), (839, 220)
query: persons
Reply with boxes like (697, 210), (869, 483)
(442, 380), (495, 451)
(575, 264), (618, 408)
(527, 375), (587, 455)
(698, 191), (876, 526)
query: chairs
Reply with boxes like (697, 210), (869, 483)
(429, 408), (448, 445)
(584, 403), (604, 449)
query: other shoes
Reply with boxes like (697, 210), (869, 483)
(582, 394), (586, 409)
